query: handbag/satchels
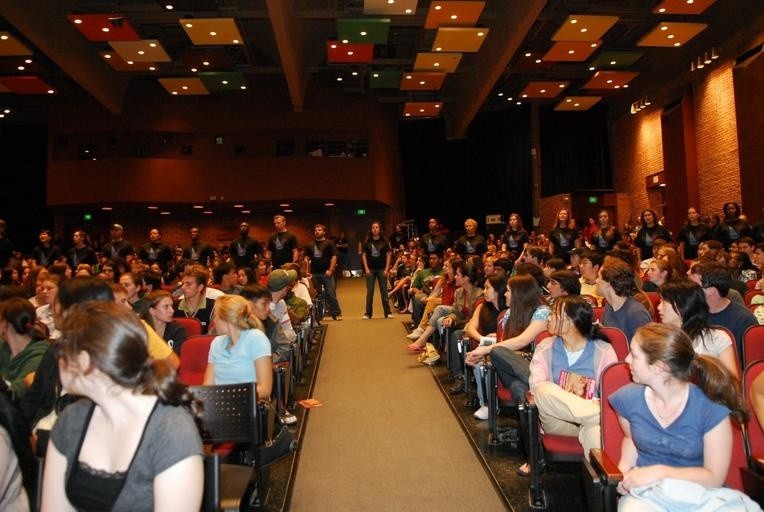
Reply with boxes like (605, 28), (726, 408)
(239, 416), (296, 468)
(417, 343), (440, 366)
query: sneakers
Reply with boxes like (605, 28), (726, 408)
(406, 329), (422, 339)
(363, 315), (373, 319)
(385, 314), (394, 318)
(280, 409), (298, 426)
(336, 315), (342, 320)
(474, 406), (500, 421)
(449, 377), (465, 393)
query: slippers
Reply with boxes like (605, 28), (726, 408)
(407, 344), (428, 351)
(517, 463), (531, 477)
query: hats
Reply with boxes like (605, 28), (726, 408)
(267, 269), (297, 291)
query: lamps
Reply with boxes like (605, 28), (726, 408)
(691, 45), (719, 72)
(630, 96), (651, 114)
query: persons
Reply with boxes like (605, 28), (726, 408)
(359, 202), (764, 511)
(0, 203), (354, 511)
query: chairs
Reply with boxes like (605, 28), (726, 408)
(440, 259), (764, 512)
(0, 286), (328, 512)
